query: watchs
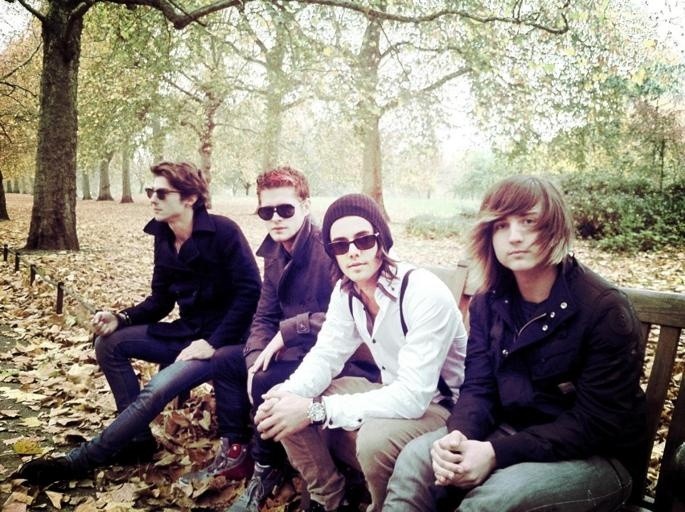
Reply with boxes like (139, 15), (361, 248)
(306, 396), (326, 425)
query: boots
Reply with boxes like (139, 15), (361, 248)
(179, 436), (254, 487)
(226, 461), (284, 512)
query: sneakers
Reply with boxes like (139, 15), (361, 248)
(301, 497), (358, 511)
(19, 457), (68, 483)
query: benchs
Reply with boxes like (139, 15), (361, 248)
(173, 259), (685, 512)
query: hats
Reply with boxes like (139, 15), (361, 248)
(321, 194), (393, 263)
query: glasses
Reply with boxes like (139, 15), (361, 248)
(257, 200), (304, 220)
(144, 188), (182, 200)
(324, 232), (379, 255)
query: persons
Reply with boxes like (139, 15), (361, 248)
(382, 176), (649, 512)
(178, 166), (383, 512)
(20, 162), (261, 486)
(253, 193), (468, 512)
